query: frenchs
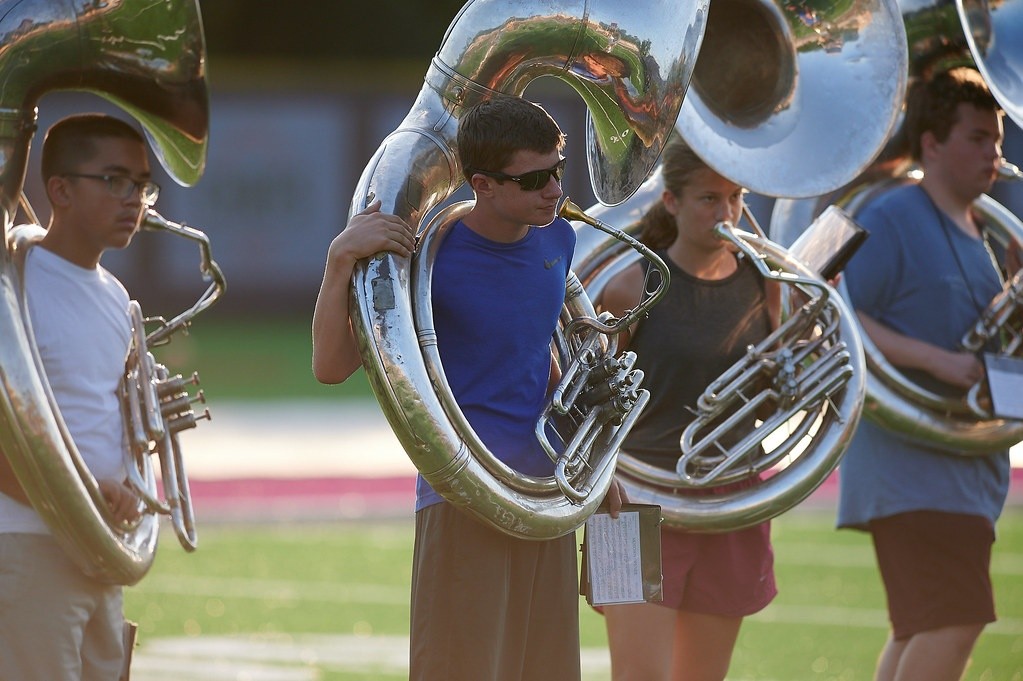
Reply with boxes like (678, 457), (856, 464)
(346, 2), (710, 541)
(771, 4), (1022, 458)
(1, 0), (228, 587)
(549, 0), (909, 535)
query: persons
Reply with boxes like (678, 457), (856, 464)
(1, 114), (160, 681)
(577, 134), (802, 681)
(313, 96), (582, 680)
(837, 67), (1023, 681)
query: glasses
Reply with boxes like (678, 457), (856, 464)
(470, 156), (567, 190)
(62, 169), (161, 206)
